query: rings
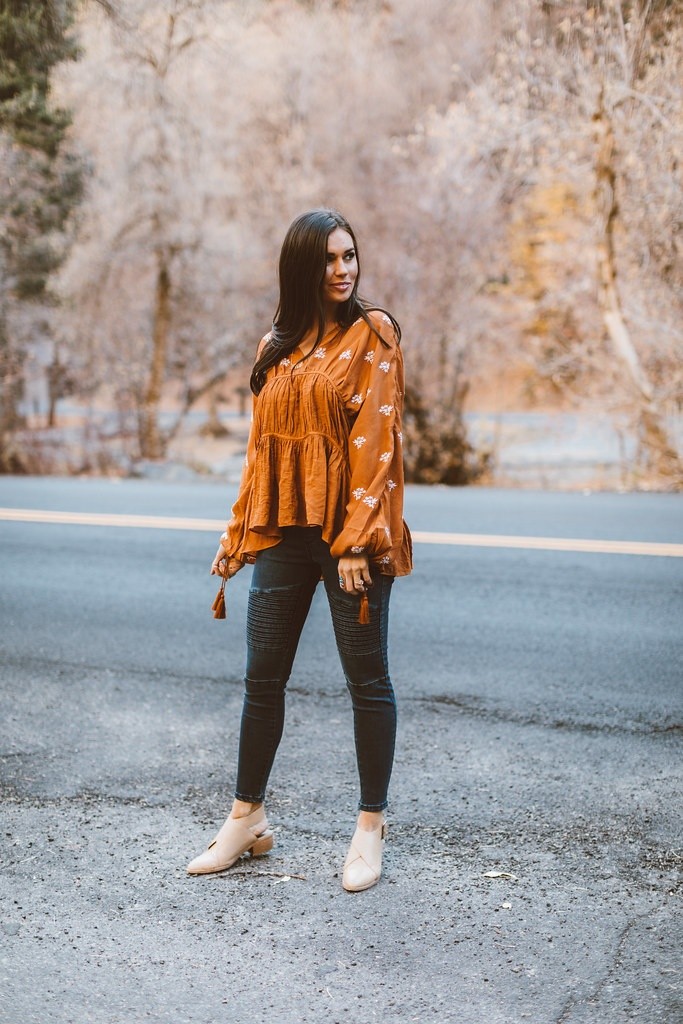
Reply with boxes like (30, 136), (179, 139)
(338, 575), (346, 589)
(354, 580), (364, 585)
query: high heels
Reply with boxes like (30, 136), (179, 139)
(342, 815), (387, 891)
(186, 805), (275, 872)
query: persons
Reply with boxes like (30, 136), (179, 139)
(183, 207), (419, 893)
